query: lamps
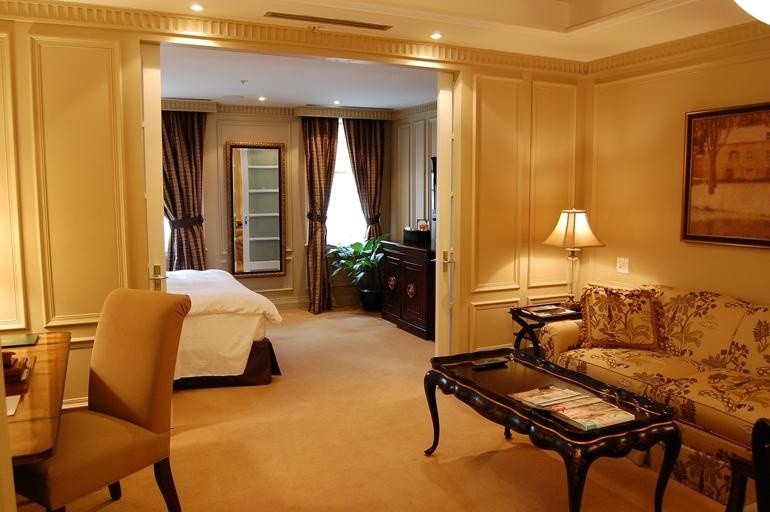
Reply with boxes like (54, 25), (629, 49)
(540, 206), (605, 310)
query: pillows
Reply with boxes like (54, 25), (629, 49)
(581, 286), (665, 351)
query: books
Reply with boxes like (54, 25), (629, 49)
(508, 383), (636, 433)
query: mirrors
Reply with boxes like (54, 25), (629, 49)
(226, 140), (286, 278)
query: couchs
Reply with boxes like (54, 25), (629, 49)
(538, 284), (769, 507)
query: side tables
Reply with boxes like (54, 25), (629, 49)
(510, 302), (583, 358)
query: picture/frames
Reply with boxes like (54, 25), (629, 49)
(681, 101), (770, 251)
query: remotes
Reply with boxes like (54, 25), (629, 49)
(471, 356), (511, 371)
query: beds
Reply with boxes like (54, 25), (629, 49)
(166, 269), (278, 385)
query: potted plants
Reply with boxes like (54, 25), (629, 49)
(326, 233), (391, 312)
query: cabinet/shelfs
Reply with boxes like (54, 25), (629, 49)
(378, 240), (435, 342)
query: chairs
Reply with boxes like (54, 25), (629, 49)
(14, 286), (192, 512)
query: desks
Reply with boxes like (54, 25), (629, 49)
(0, 332), (70, 464)
